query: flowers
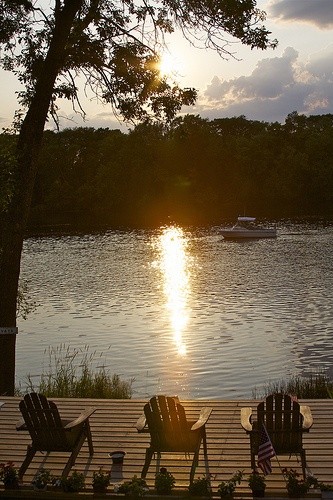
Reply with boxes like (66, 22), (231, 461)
(155, 467), (176, 495)
(114, 475), (149, 496)
(91, 465), (111, 495)
(0, 462), (86, 491)
(187, 473), (213, 500)
(248, 468), (266, 490)
(217, 469), (245, 496)
(282, 465), (333, 492)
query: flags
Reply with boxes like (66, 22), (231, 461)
(258, 425), (275, 476)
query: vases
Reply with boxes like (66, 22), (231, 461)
(160, 488), (169, 495)
(64, 486), (75, 492)
(125, 491), (137, 500)
(95, 489), (105, 497)
(4, 477), (18, 488)
(289, 489), (307, 498)
(108, 450), (126, 464)
(222, 495), (233, 500)
(322, 491), (333, 499)
(253, 490), (264, 497)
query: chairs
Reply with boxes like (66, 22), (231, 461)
(17, 392), (97, 479)
(136, 395), (213, 484)
(241, 392), (315, 481)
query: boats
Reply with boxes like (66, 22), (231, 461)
(217, 217), (282, 239)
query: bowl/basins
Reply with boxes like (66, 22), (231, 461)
(107, 450), (127, 463)
